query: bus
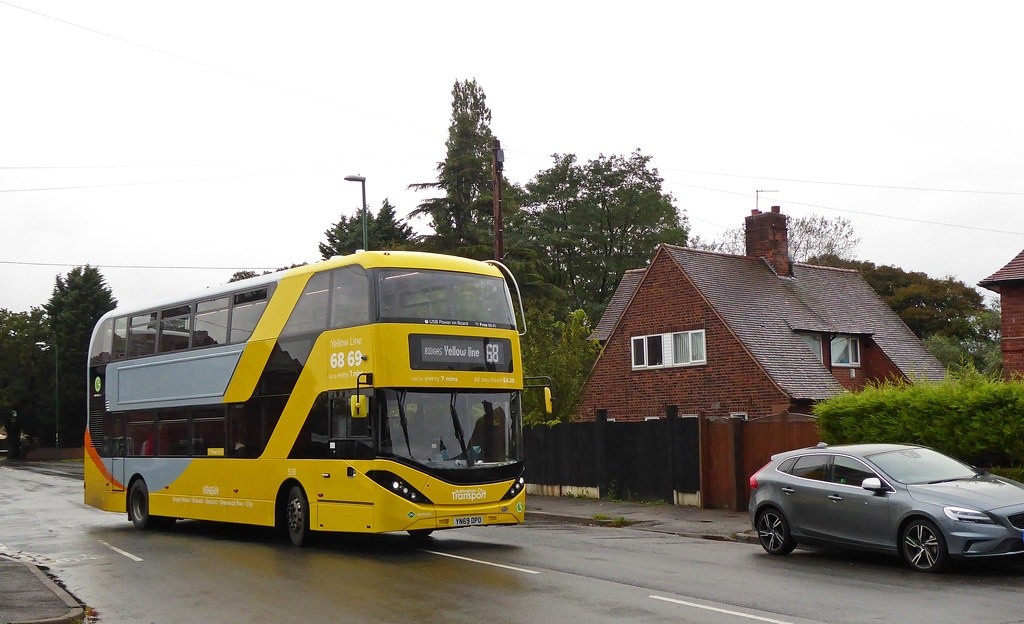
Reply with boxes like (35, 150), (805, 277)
(83, 248), (556, 548)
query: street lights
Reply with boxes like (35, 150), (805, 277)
(344, 174), (369, 250)
(35, 341), (61, 460)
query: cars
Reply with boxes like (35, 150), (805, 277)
(747, 441), (1024, 574)
